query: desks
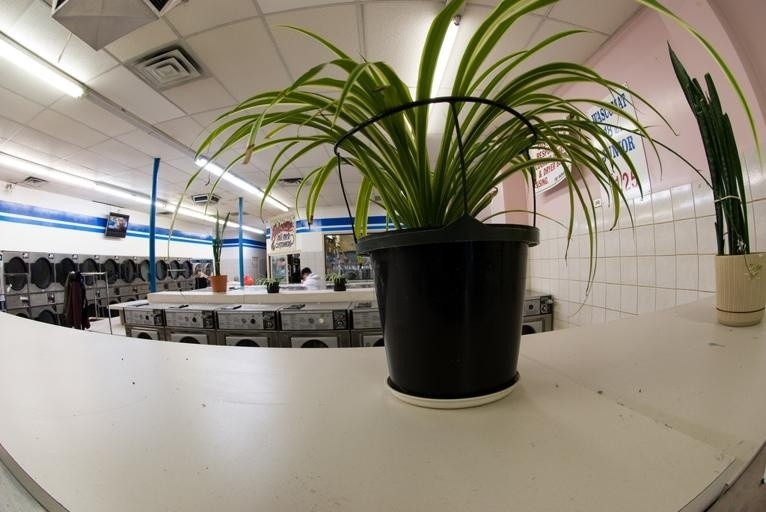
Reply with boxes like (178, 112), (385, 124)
(230, 282), (375, 290)
(2, 310), (736, 512)
(520, 299), (766, 510)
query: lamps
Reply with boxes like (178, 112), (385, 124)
(194, 156), (289, 212)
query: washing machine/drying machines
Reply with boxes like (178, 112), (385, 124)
(1, 250), (216, 332)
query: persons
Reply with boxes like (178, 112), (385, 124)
(301, 266), (328, 290)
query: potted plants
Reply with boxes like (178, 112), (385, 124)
(166, 0), (714, 411)
(262, 278), (279, 293)
(322, 269), (352, 291)
(207, 207), (231, 292)
(666, 40), (766, 327)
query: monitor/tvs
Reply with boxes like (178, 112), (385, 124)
(105, 213), (128, 239)
